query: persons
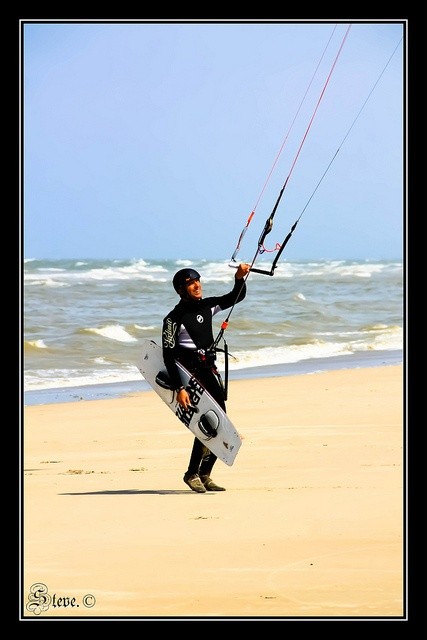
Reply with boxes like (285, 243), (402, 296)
(162, 264), (251, 493)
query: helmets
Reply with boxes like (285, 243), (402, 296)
(173, 269), (201, 295)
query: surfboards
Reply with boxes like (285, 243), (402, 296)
(135, 337), (243, 468)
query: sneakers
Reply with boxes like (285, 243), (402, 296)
(198, 473), (225, 491)
(184, 471), (206, 493)
